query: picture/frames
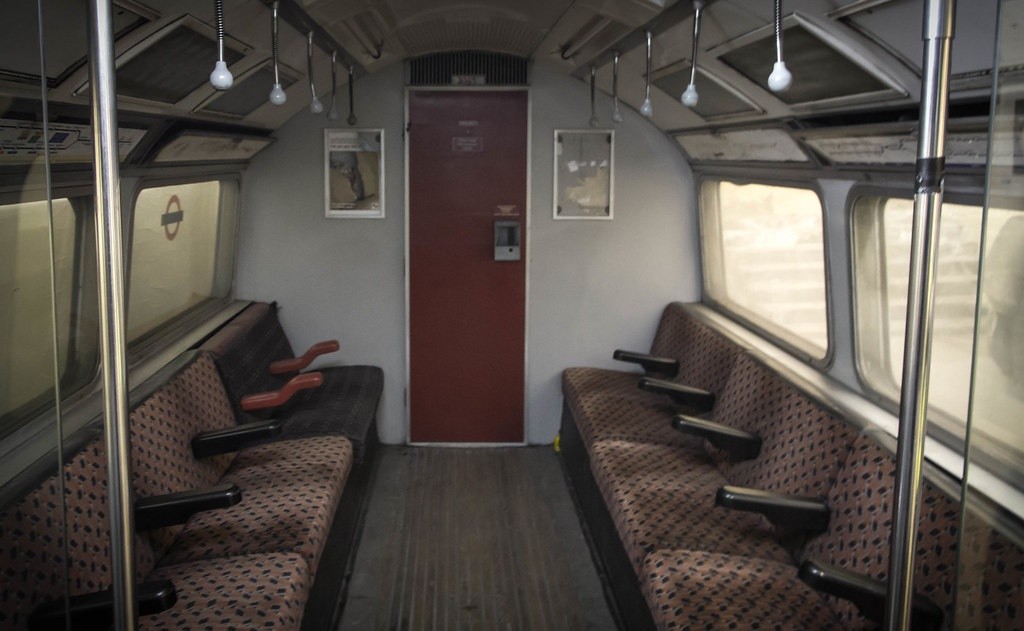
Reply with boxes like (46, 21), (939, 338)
(552, 128), (615, 220)
(323, 127), (386, 219)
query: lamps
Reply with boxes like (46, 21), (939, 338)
(589, 63), (600, 129)
(639, 29), (654, 117)
(306, 31), (323, 114)
(611, 49), (624, 124)
(767, 0), (793, 95)
(268, 0), (288, 106)
(210, 0), (233, 90)
(681, 0), (703, 107)
(327, 50), (339, 122)
(346, 64), (357, 127)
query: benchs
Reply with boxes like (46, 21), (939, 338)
(555, 299), (1023, 631)
(0, 303), (383, 631)
